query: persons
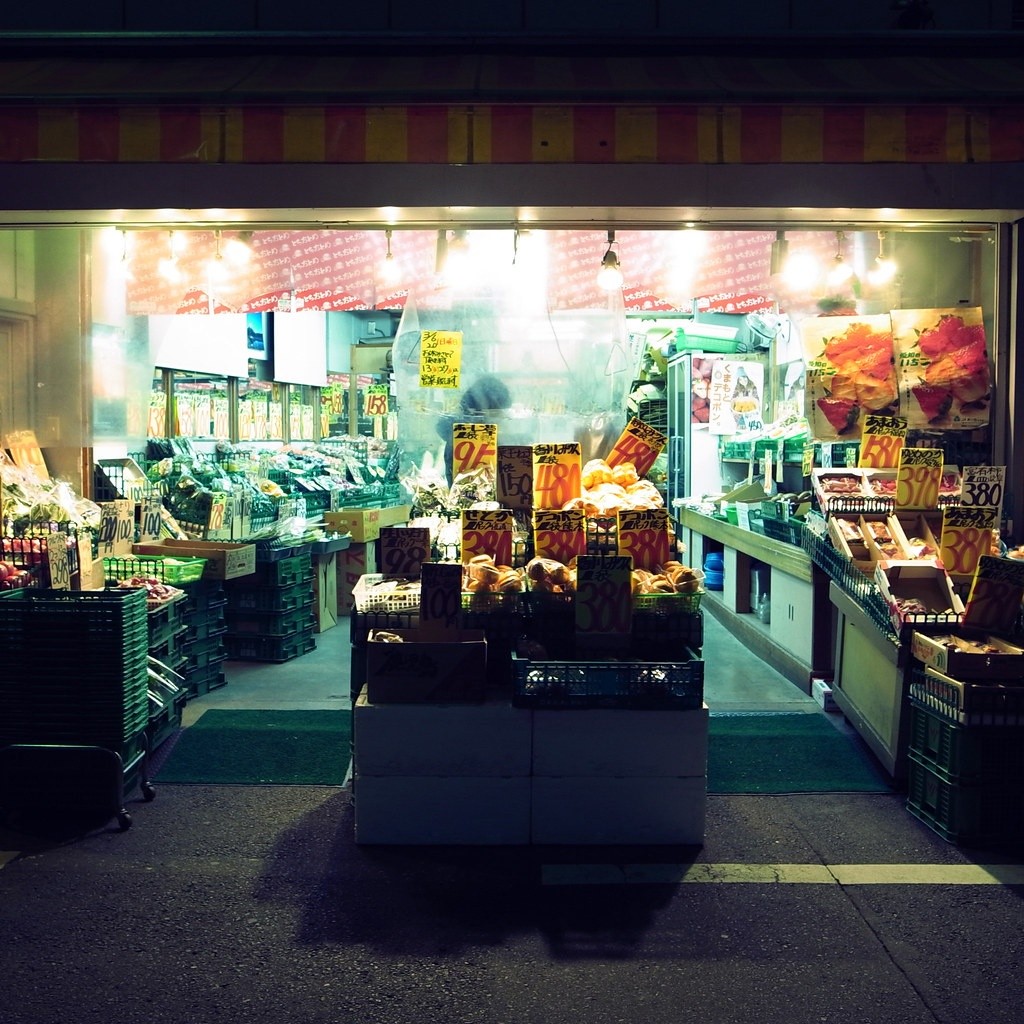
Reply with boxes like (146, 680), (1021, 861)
(434, 377), (509, 493)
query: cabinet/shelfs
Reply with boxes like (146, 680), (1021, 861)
(680, 507), (832, 697)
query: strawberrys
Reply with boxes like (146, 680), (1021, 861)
(816, 322), (897, 434)
(690, 359), (713, 424)
(910, 315), (992, 423)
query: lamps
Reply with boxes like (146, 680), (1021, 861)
(597, 230), (624, 291)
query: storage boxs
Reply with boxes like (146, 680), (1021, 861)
(354, 683), (709, 845)
(325, 510), (380, 543)
(96, 457), (156, 500)
(511, 640), (704, 710)
(812, 678), (840, 712)
(336, 541), (375, 616)
(367, 628), (491, 703)
(132, 538), (256, 580)
(0, 588), (149, 810)
(311, 553), (338, 633)
(713, 481), (811, 532)
(811, 465), (1024, 847)
(147, 580), (228, 752)
(227, 553), (317, 664)
